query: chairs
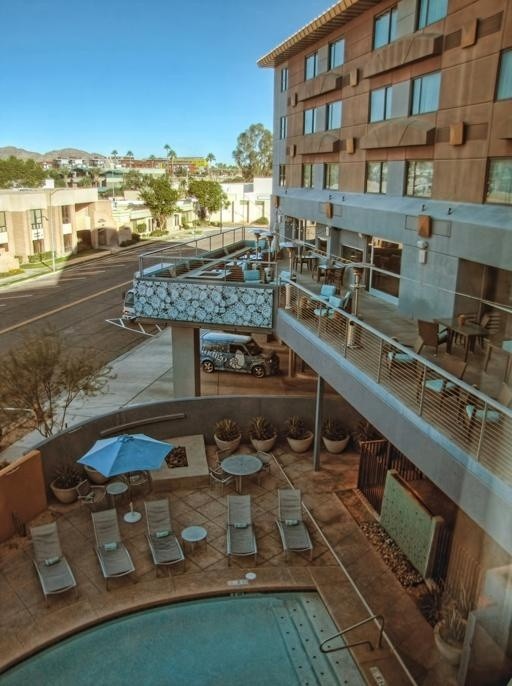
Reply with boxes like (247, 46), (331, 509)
(208, 466), (239, 496)
(30, 522), (79, 608)
(468, 314), (492, 350)
(415, 318), (448, 359)
(118, 470), (150, 499)
(143, 497), (185, 578)
(382, 337), (417, 379)
(92, 509), (137, 592)
(502, 340), (512, 352)
(416, 351), (467, 411)
(215, 448), (234, 466)
(456, 381), (512, 441)
(252, 451), (272, 475)
(77, 479), (108, 512)
(276, 489), (314, 563)
(224, 494), (258, 568)
(215, 242), (352, 331)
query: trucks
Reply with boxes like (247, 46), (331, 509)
(122, 289), (136, 322)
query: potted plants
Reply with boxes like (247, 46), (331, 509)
(247, 416), (278, 453)
(48, 460), (82, 504)
(449, 580), (477, 630)
(352, 420), (383, 456)
(320, 415), (350, 454)
(282, 416), (315, 452)
(212, 419), (242, 453)
(435, 610), (469, 665)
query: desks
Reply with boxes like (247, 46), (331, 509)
(220, 456), (263, 495)
(186, 270), (232, 283)
(182, 528), (208, 557)
(432, 318), (496, 362)
(106, 482), (128, 508)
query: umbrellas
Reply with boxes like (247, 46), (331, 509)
(77, 433), (173, 513)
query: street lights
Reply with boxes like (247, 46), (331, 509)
(48, 187), (75, 273)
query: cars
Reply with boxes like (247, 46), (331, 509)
(199, 330), (279, 377)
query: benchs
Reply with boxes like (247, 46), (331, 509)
(154, 240), (250, 280)
